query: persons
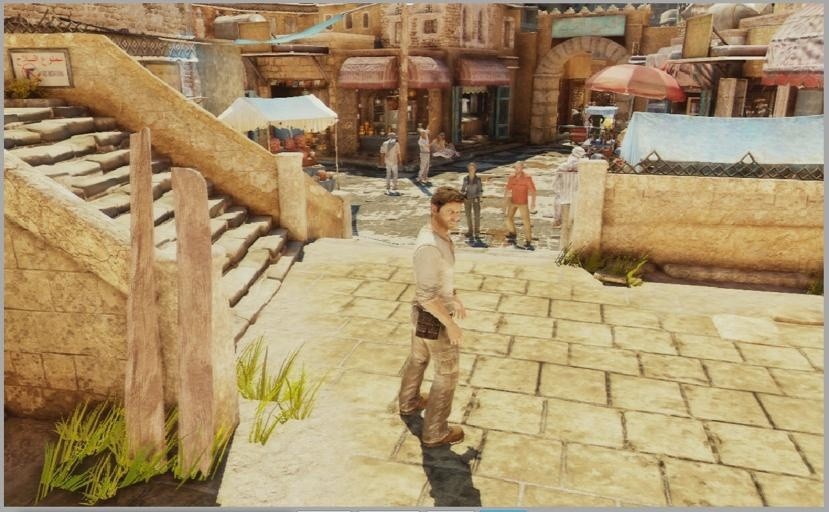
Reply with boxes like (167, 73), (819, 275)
(397, 185), (471, 448)
(501, 160), (538, 247)
(430, 131), (464, 160)
(414, 116), (439, 187)
(460, 163), (485, 239)
(380, 132), (403, 196)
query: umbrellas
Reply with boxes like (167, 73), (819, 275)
(584, 63), (688, 123)
